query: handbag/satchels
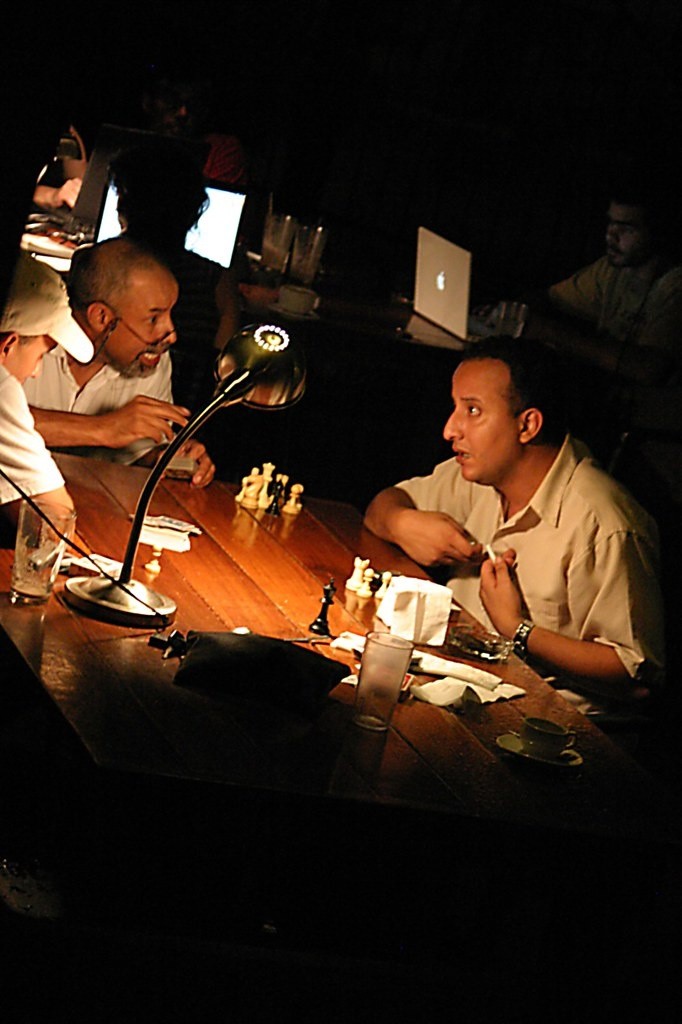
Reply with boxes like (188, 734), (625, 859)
(175, 630), (350, 726)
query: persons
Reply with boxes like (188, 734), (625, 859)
(362, 345), (667, 770)
(41, 85), (250, 423)
(1, 251), (75, 536)
(477, 190), (682, 512)
(29, 238), (217, 488)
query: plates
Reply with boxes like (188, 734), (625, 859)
(449, 625), (514, 660)
(496, 735), (583, 766)
(267, 304), (320, 320)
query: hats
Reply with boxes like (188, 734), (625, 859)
(0, 253), (95, 363)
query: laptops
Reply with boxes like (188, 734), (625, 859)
(412, 226), (502, 342)
(47, 124), (211, 235)
(93, 156), (251, 273)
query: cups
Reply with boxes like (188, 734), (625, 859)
(520, 717), (578, 759)
(11, 499), (76, 603)
(280, 285), (320, 313)
(260, 215), (296, 275)
(353, 632), (414, 730)
(291, 226), (328, 284)
(496, 302), (527, 344)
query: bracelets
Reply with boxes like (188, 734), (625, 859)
(512, 621), (536, 656)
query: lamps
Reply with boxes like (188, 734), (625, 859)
(64, 323), (305, 629)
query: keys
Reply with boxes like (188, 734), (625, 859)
(163, 630), (186, 659)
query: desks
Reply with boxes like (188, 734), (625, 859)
(0, 451), (682, 1024)
(179, 251), (470, 516)
